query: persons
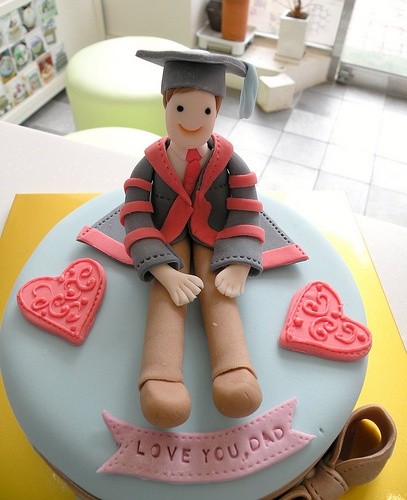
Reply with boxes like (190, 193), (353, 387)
(77, 49), (309, 430)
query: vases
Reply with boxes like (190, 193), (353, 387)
(277, 14), (311, 60)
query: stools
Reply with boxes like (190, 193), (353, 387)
(65, 36), (192, 139)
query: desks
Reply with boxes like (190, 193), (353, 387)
(0, 122), (406, 350)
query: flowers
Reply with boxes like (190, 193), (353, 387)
(277, 0), (312, 18)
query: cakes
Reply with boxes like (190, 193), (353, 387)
(0, 48), (397, 500)
